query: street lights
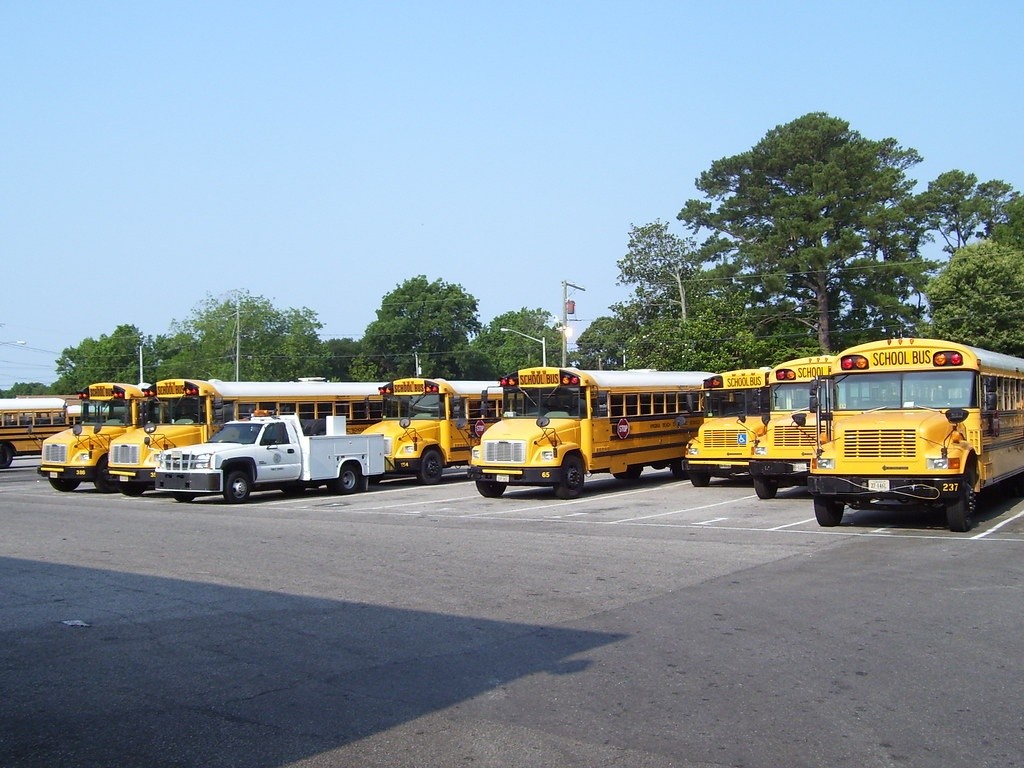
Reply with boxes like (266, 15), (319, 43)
(139, 341), (147, 384)
(500, 327), (546, 366)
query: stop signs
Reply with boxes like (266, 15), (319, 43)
(474, 420), (487, 438)
(617, 419), (630, 440)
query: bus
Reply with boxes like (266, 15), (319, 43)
(465, 366), (720, 500)
(675, 366), (792, 486)
(35, 382), (152, 493)
(103, 378), (408, 497)
(735, 354), (850, 499)
(0, 398), (71, 469)
(357, 377), (525, 485)
(808, 337), (1024, 533)
(59, 405), (126, 432)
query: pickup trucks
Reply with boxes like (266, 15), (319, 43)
(153, 409), (386, 504)
